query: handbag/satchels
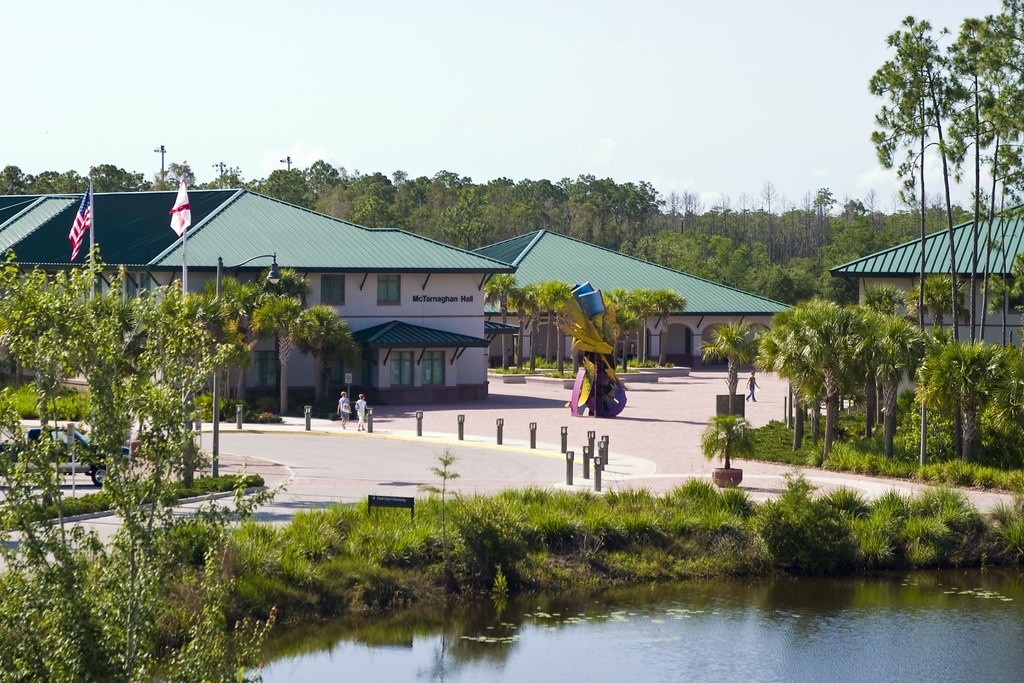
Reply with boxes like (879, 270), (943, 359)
(343, 398), (351, 413)
(355, 404), (358, 410)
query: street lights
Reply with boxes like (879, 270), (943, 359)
(213, 250), (282, 479)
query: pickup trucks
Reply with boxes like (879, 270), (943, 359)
(1, 427), (130, 487)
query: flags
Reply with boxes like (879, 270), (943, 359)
(68, 186), (90, 260)
(169, 182), (190, 238)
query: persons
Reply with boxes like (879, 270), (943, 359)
(746, 372), (759, 402)
(337, 392), (352, 429)
(355, 394), (369, 432)
